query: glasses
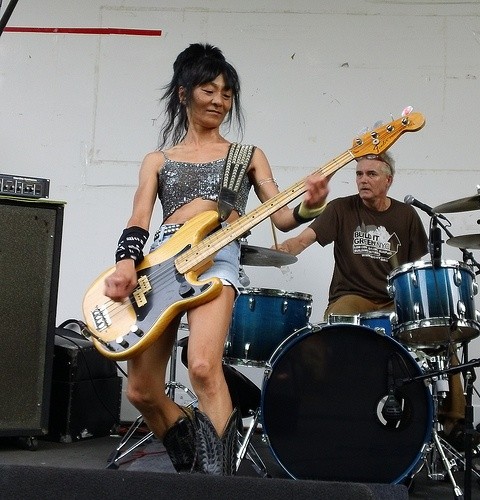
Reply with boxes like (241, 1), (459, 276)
(354, 155), (393, 167)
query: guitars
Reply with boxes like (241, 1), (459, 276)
(82, 111), (426, 359)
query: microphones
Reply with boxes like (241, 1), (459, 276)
(405, 195), (447, 220)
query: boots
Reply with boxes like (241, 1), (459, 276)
(193, 407), (238, 474)
(159, 416), (199, 474)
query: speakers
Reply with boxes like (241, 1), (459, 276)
(0, 464), (409, 500)
(50, 333), (122, 444)
(0, 198), (63, 435)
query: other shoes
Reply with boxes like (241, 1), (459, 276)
(441, 424), (479, 450)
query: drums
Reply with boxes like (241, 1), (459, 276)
(218, 288), (312, 369)
(260, 323), (435, 485)
(387, 260), (480, 342)
(328, 310), (394, 341)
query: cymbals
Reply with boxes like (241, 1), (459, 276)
(432, 195), (480, 214)
(239, 243), (297, 267)
(448, 233), (480, 251)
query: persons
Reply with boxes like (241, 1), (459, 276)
(273, 151), (480, 453)
(105, 43), (330, 477)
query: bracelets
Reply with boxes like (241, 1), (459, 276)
(116, 226), (149, 267)
(293, 201), (327, 224)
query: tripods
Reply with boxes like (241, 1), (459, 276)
(403, 351), (479, 496)
(107, 325), (200, 469)
